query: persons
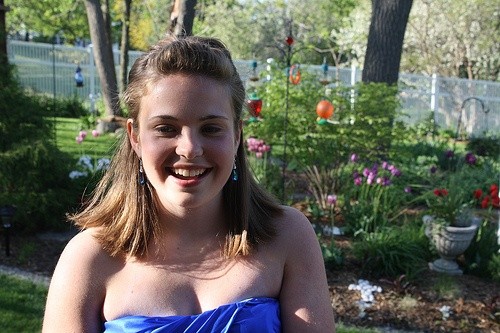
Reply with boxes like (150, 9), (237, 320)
(42, 33), (335, 333)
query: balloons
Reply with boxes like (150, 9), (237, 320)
(316, 100), (333, 119)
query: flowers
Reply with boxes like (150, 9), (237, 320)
(421, 160), (500, 227)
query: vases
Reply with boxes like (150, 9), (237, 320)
(423, 215), (480, 257)
